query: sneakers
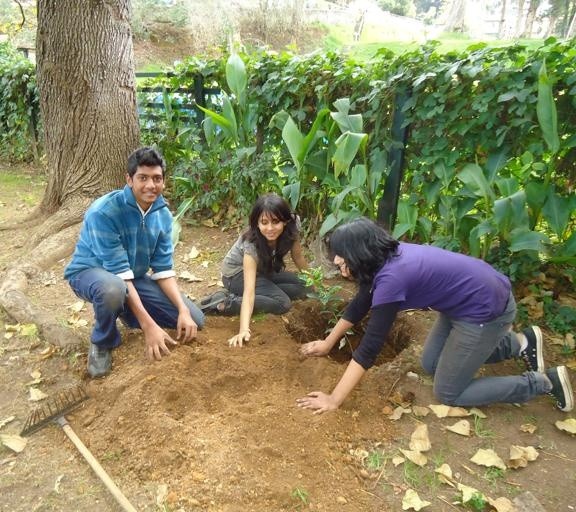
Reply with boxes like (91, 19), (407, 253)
(201, 290), (226, 315)
(86, 344), (113, 378)
(520, 326), (545, 373)
(545, 366), (574, 412)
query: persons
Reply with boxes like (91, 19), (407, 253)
(64, 147), (206, 378)
(296, 217), (573, 416)
(198, 194), (316, 348)
